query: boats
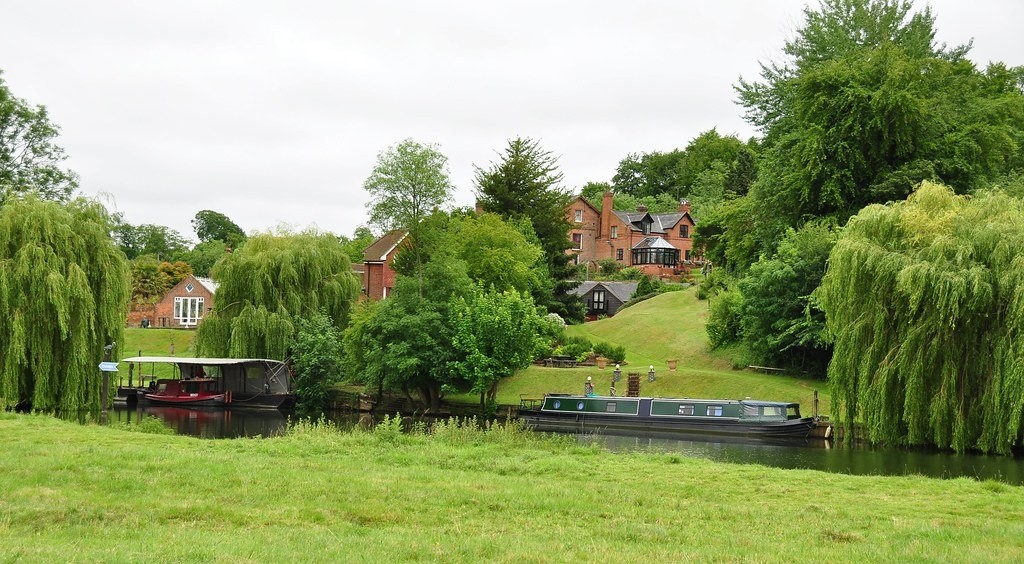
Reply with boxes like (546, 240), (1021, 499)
(511, 391), (821, 440)
(111, 349), (297, 411)
(134, 374), (233, 408)
